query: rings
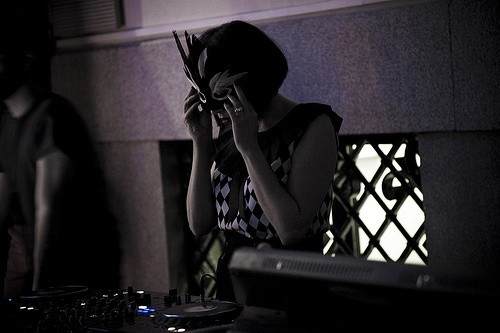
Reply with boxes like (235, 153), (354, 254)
(235, 107), (243, 113)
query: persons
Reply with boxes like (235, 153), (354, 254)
(183, 22), (343, 302)
(1, 47), (124, 293)
